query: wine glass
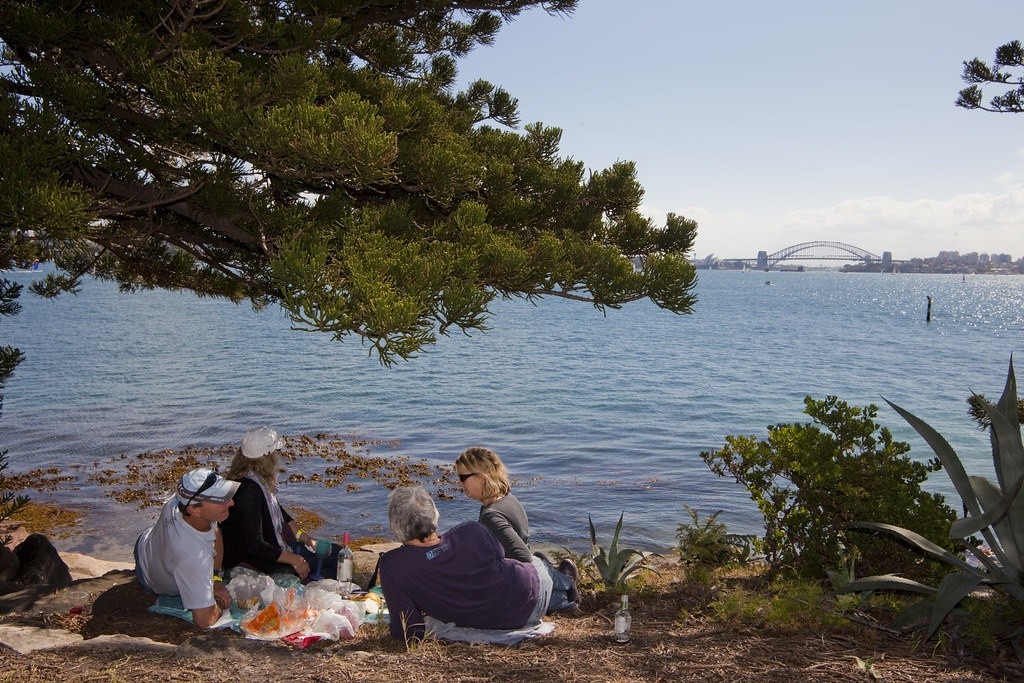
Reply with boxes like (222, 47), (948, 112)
(308, 538), (331, 581)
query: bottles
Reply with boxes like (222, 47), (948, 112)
(337, 532), (354, 599)
(614, 594), (631, 643)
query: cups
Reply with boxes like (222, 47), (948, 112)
(69, 591), (88, 618)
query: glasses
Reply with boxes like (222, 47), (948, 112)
(458, 473), (480, 483)
(185, 472), (218, 511)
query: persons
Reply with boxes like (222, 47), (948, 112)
(133, 467), (241, 629)
(217, 425), (343, 586)
(455, 447), (533, 563)
(379, 486), (580, 642)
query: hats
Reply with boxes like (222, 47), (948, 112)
(176, 468), (242, 506)
(241, 428), (286, 459)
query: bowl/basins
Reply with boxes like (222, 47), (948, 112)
(235, 584), (266, 609)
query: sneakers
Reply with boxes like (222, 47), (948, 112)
(533, 550), (554, 566)
(559, 558), (580, 603)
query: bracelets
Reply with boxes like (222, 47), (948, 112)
(295, 528), (305, 542)
(213, 570), (223, 582)
